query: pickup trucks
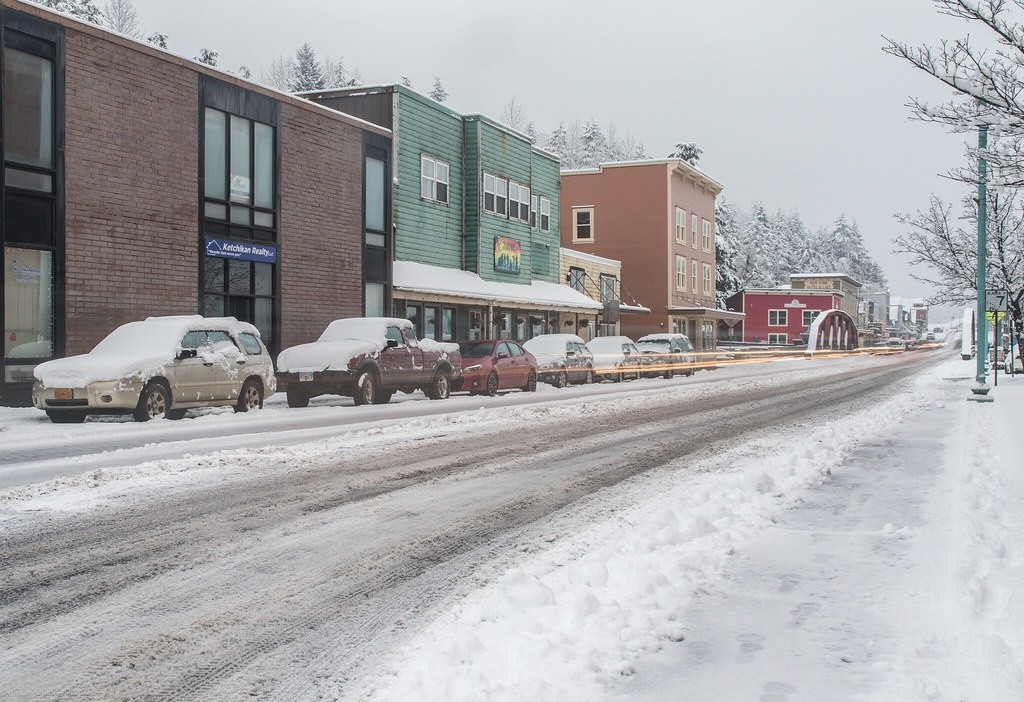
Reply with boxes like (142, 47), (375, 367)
(276, 317), (464, 408)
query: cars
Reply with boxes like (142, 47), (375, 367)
(933, 327), (943, 333)
(451, 338), (540, 396)
(8, 340), (52, 380)
(1005, 354), (1024, 374)
(32, 317), (279, 423)
(926, 332), (936, 340)
(585, 336), (643, 383)
(636, 332), (696, 379)
(522, 335), (596, 388)
